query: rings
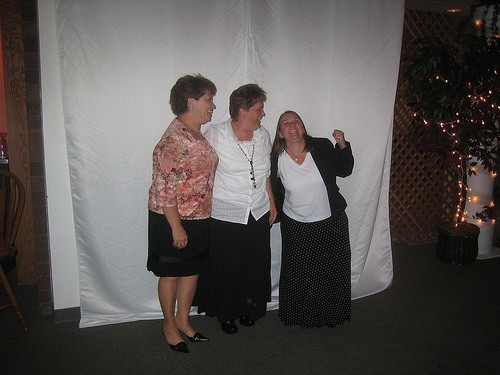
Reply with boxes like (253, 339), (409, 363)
(178, 242), (181, 246)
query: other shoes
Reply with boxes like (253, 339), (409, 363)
(240, 316), (255, 326)
(222, 317), (238, 334)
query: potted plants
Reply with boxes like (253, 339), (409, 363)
(403, 34), (500, 266)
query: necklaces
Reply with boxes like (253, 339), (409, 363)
(286, 144), (305, 161)
(233, 135), (256, 189)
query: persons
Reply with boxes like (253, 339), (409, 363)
(146, 73), (219, 354)
(270, 111), (354, 328)
(192, 84), (277, 334)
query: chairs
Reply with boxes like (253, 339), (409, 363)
(0, 170), (30, 332)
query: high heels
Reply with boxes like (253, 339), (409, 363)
(177, 326), (209, 342)
(162, 326), (190, 354)
